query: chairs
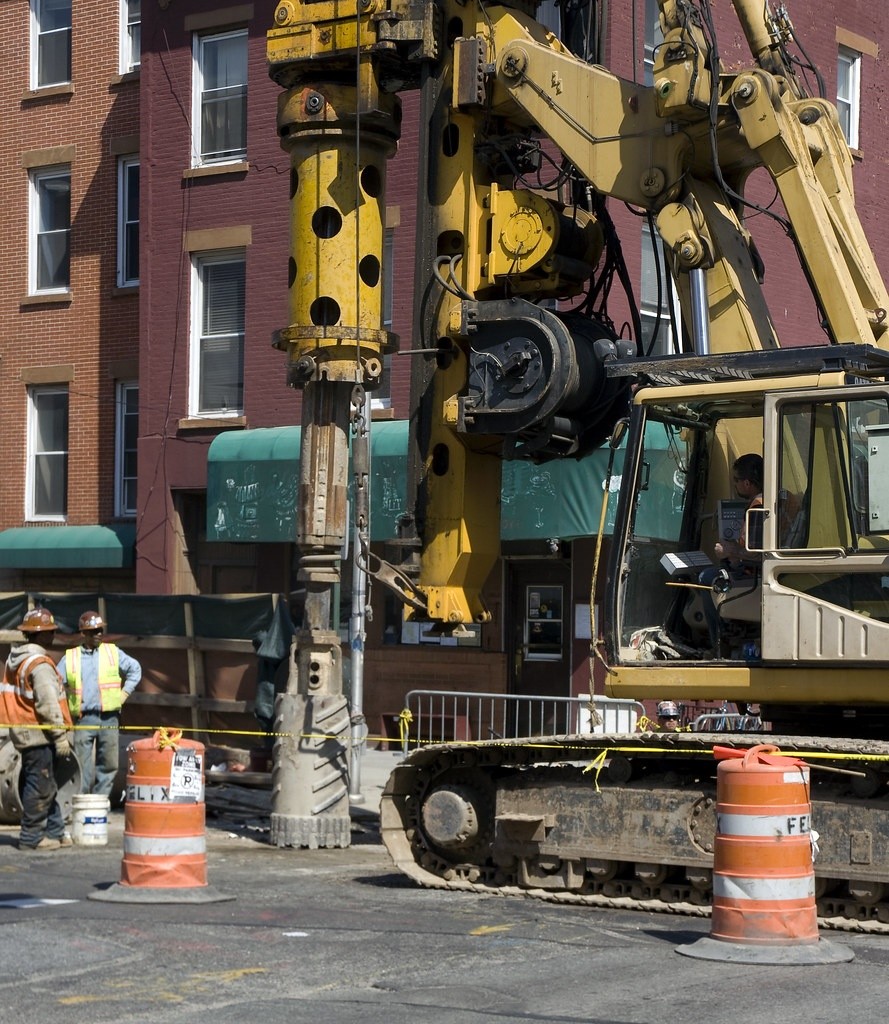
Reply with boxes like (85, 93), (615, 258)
(710, 487), (807, 659)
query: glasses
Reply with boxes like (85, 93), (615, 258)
(733, 476), (745, 483)
(660, 716), (678, 720)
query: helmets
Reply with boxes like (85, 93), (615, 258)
(78, 611), (107, 631)
(656, 701), (680, 716)
(17, 608), (58, 631)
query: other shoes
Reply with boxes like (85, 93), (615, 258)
(18, 836), (60, 850)
(702, 647), (728, 658)
(57, 837), (72, 847)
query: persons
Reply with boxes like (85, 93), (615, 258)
(54, 609), (145, 823)
(0, 606), (74, 851)
(698, 450), (801, 659)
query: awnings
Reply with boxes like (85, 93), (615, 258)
(205, 417), (690, 540)
(0, 524), (136, 568)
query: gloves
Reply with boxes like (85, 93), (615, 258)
(56, 739), (71, 758)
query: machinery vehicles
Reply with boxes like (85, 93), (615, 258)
(1, 0), (889, 945)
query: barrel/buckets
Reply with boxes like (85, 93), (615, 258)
(71, 794), (110, 845)
